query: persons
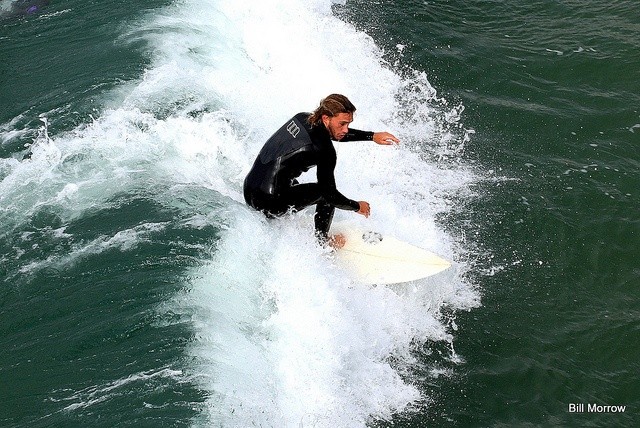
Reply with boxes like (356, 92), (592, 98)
(242, 93), (401, 258)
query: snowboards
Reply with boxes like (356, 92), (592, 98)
(338, 231), (452, 285)
